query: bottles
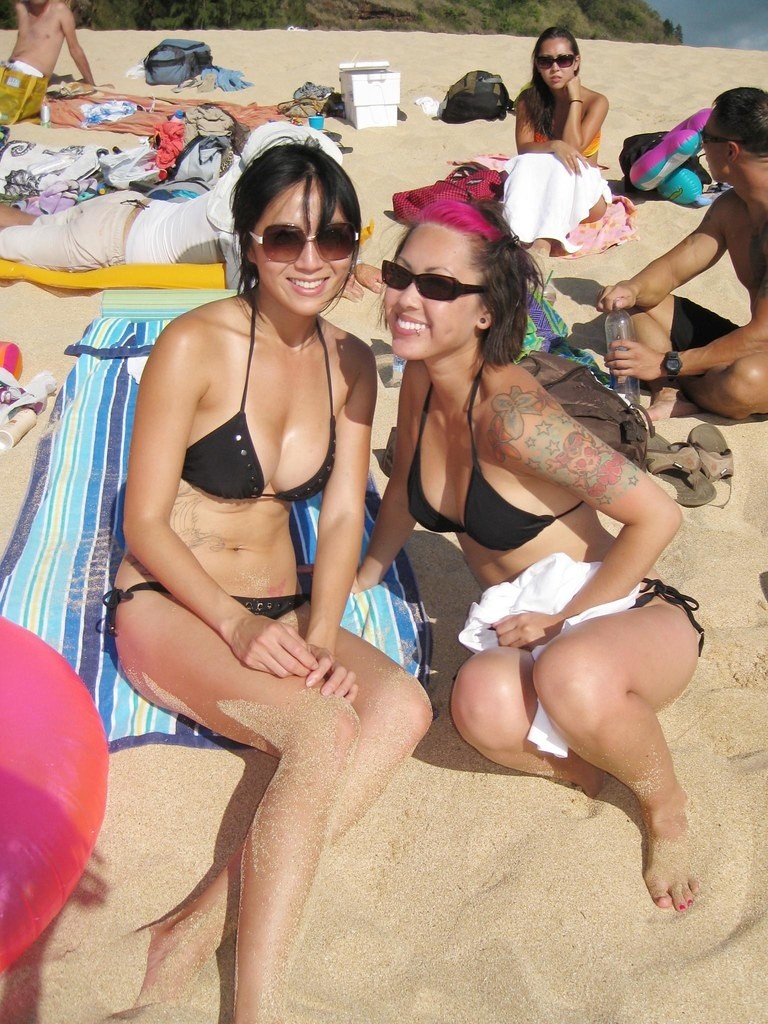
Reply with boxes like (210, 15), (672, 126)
(169, 110), (183, 122)
(391, 352), (408, 382)
(0, 408), (36, 449)
(604, 308), (641, 407)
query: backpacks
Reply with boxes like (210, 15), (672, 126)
(438, 70), (514, 121)
(520, 348), (653, 476)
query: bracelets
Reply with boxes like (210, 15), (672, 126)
(569, 99), (583, 104)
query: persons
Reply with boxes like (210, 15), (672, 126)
(515, 27), (607, 259)
(0, 0), (115, 125)
(102, 134), (438, 1024)
(595, 86), (768, 421)
(0, 121), (393, 303)
(297, 188), (701, 912)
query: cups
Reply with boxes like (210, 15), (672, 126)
(533, 284), (555, 306)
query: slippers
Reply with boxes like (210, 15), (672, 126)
(688, 424), (733, 509)
(642, 429), (716, 508)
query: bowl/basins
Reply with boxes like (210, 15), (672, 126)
(309, 116), (324, 130)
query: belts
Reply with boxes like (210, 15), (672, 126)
(122, 196), (154, 252)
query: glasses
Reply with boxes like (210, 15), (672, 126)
(250, 223), (359, 263)
(381, 259), (488, 302)
(699, 125), (742, 147)
(535, 54), (577, 70)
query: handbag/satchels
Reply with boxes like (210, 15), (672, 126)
(276, 95), (327, 118)
(391, 162), (508, 228)
(143, 38), (214, 85)
(619, 131), (721, 193)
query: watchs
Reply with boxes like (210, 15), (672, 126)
(664, 351), (682, 381)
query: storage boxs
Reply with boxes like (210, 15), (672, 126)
(339, 60), (404, 130)
